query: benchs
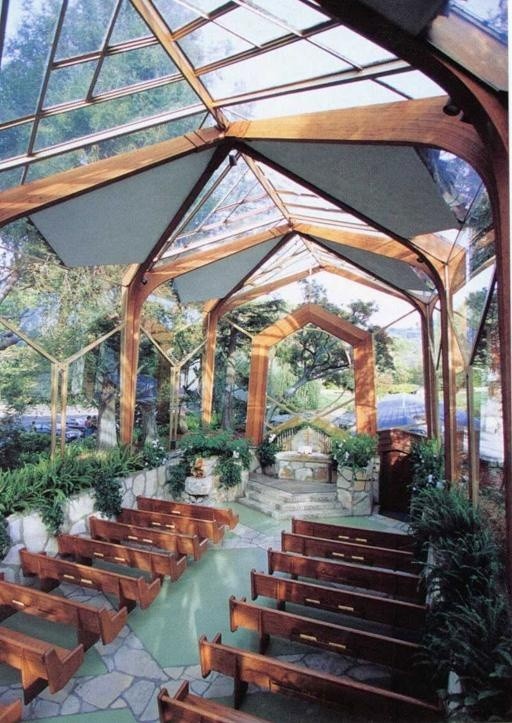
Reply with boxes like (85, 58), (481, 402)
(267, 547), (425, 606)
(57, 532), (187, 586)
(0, 572), (128, 653)
(116, 507), (225, 544)
(200, 633), (444, 723)
(17, 547), (161, 614)
(249, 568), (429, 642)
(157, 679), (271, 723)
(89, 516), (209, 562)
(229, 595), (423, 691)
(280, 530), (422, 577)
(136, 494), (239, 530)
(290, 516), (418, 551)
(0, 625), (84, 706)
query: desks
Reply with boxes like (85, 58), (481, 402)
(275, 451), (332, 482)
